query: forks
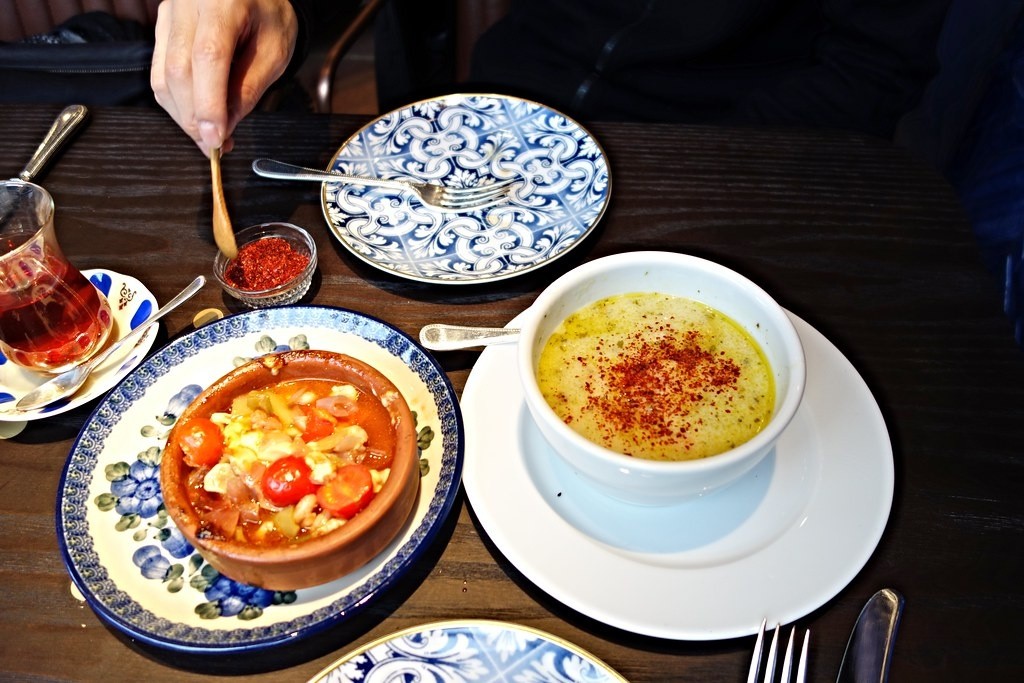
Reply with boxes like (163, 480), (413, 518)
(747, 617), (812, 683)
(251, 158), (514, 212)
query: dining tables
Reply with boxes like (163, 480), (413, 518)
(0, 103), (1023, 683)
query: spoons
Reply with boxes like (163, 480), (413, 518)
(210, 149), (237, 259)
(15, 274), (207, 411)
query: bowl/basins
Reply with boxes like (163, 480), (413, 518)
(518, 250), (809, 493)
(213, 221), (317, 309)
(158, 349), (419, 589)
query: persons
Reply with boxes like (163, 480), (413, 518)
(149, 0), (1024, 354)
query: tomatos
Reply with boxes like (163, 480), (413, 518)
(260, 454), (320, 507)
(316, 465), (374, 517)
(176, 417), (226, 468)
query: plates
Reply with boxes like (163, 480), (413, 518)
(55, 305), (466, 653)
(459, 305), (896, 641)
(320, 91), (614, 285)
(0, 268), (159, 423)
(305, 619), (630, 681)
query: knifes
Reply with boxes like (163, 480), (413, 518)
(0, 104), (87, 218)
(838, 589), (898, 683)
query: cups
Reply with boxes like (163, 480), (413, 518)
(0, 178), (115, 373)
